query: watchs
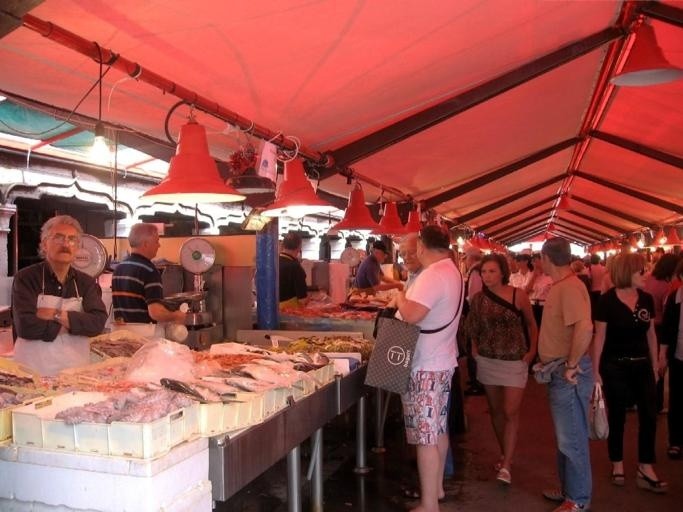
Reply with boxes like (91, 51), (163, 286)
(53, 308), (62, 322)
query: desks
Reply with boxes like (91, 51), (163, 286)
(210, 320), (387, 512)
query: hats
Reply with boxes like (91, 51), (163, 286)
(370, 240), (392, 256)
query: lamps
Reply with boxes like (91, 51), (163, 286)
(608, 14), (683, 86)
(90, 59), (110, 164)
(226, 136), (277, 194)
(331, 179), (458, 244)
(259, 150), (339, 219)
(587, 222), (683, 253)
(138, 105), (247, 202)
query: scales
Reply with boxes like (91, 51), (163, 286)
(71, 234), (111, 334)
(163, 236), (217, 330)
(340, 247), (361, 283)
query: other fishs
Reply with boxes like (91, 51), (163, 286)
(159, 352), (329, 405)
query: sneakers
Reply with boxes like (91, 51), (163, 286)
(551, 499), (593, 512)
(667, 445), (681, 459)
(541, 487), (565, 500)
(494, 462), (511, 484)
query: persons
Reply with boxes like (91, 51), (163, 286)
(279, 233), (307, 302)
(112, 222), (186, 336)
(12, 213), (109, 377)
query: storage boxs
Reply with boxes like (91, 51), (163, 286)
(0, 481), (213, 512)
(0, 438), (213, 512)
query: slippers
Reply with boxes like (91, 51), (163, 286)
(401, 486), (446, 502)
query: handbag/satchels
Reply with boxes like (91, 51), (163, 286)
(364, 299), (421, 395)
(531, 360), (563, 384)
(586, 382), (610, 441)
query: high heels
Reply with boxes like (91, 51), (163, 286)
(611, 465), (625, 486)
(635, 466), (668, 493)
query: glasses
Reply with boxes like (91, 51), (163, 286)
(45, 235), (81, 245)
